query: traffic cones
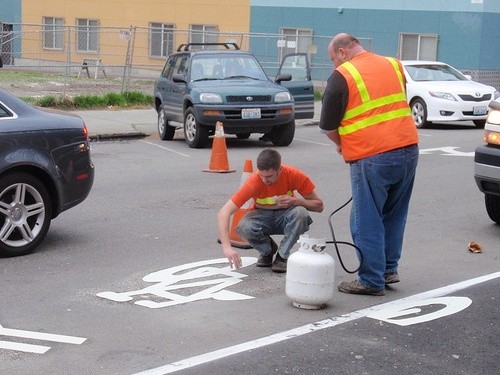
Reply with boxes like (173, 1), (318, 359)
(201, 121), (236, 173)
(218, 160), (254, 248)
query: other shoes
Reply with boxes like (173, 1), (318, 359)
(384, 272), (400, 284)
(272, 252), (287, 272)
(257, 241), (278, 266)
(338, 279), (385, 296)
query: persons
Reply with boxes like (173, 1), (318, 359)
(217, 148), (324, 273)
(319, 32), (419, 296)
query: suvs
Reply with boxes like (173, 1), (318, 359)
(153, 42), (315, 148)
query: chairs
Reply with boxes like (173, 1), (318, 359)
(192, 63), (203, 80)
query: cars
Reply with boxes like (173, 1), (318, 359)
(0, 90), (94, 256)
(476, 97), (500, 223)
(399, 61), (499, 129)
